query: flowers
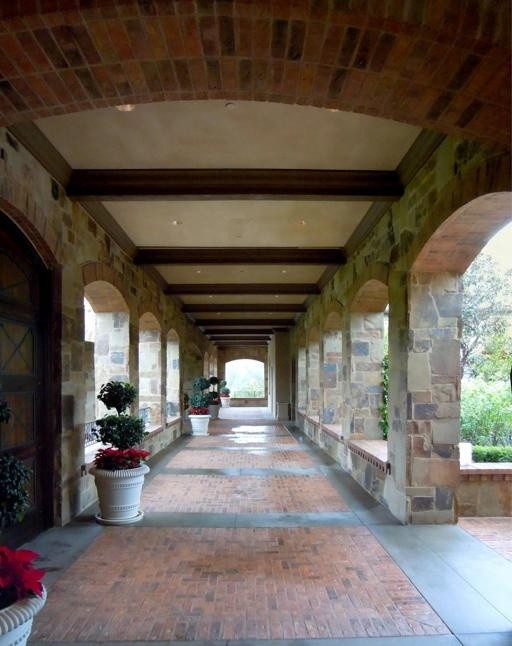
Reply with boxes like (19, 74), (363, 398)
(222, 393), (229, 397)
(214, 400), (220, 405)
(0, 547), (46, 600)
(93, 447), (150, 472)
(188, 407), (209, 415)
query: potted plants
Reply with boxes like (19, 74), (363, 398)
(208, 376), (220, 419)
(188, 376), (211, 435)
(89, 381), (151, 525)
(0, 395), (48, 646)
(220, 380), (230, 407)
